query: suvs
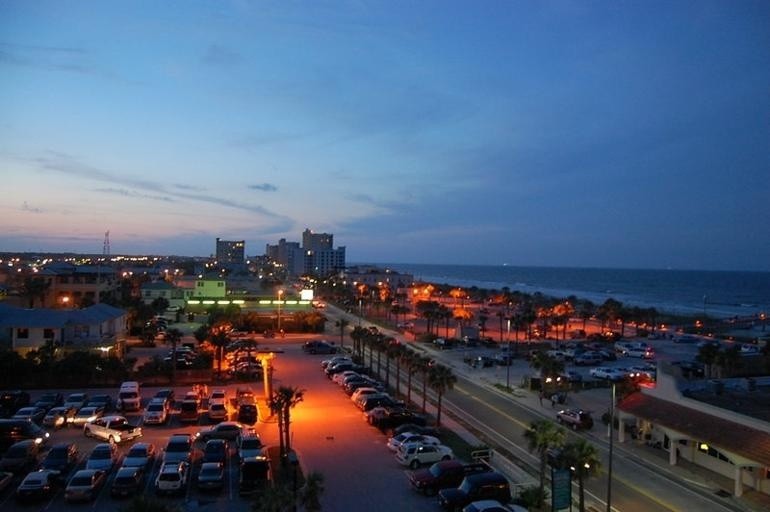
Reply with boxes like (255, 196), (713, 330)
(482, 336), (497, 347)
(741, 344), (760, 353)
(547, 446), (590, 480)
(460, 335), (480, 347)
(496, 353), (512, 366)
(557, 409), (593, 431)
(433, 338), (452, 350)
(528, 349), (544, 360)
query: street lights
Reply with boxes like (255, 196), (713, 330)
(277, 290), (283, 329)
(356, 299), (363, 354)
(605, 377), (657, 512)
(703, 295), (707, 329)
(505, 314), (514, 391)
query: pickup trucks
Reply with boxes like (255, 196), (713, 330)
(225, 329), (249, 336)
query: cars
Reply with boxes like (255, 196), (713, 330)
(320, 356), (529, 512)
(226, 339), (263, 378)
(648, 333), (661, 339)
(1, 381), (275, 503)
(397, 321), (414, 329)
(166, 304), (180, 312)
(145, 315), (175, 333)
(548, 328), (655, 378)
(263, 329), (286, 339)
(499, 343), (510, 350)
(303, 341), (340, 354)
(165, 343), (199, 368)
(672, 334), (700, 344)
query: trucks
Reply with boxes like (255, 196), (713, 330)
(464, 350), (495, 369)
